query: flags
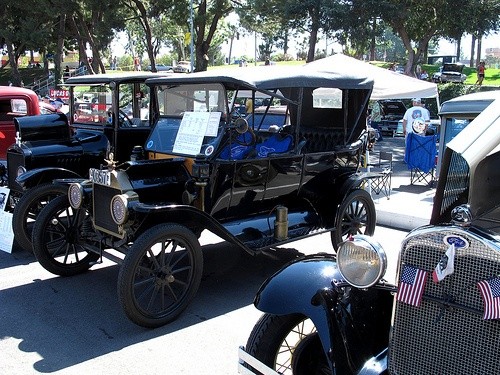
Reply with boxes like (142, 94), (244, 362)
(432, 242), (455, 282)
(478, 276), (500, 319)
(396, 265), (429, 307)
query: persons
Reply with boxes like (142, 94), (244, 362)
(65, 65), (70, 76)
(420, 70), (428, 79)
(38, 93), (70, 114)
(403, 97), (430, 146)
(475, 61), (488, 85)
(109, 55), (117, 70)
(132, 57), (140, 71)
(8, 80), (24, 88)
(245, 98), (253, 113)
(27, 62), (41, 68)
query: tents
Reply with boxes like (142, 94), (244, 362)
(301, 53), (440, 121)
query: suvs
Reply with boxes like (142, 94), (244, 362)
(174, 60), (193, 74)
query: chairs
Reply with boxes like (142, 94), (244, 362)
(403, 131), (439, 189)
(126, 118), (143, 127)
(220, 127), (295, 160)
(393, 123), (405, 138)
(370, 151), (393, 200)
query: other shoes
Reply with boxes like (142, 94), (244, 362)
(376, 137), (383, 142)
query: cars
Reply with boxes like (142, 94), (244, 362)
(148, 63), (174, 72)
(31, 64), (378, 329)
(236, 90), (500, 375)
(0, 84), (62, 161)
(431, 62), (465, 85)
(433, 89), (500, 181)
(243, 105), (290, 130)
(371, 99), (408, 137)
(0, 70), (167, 256)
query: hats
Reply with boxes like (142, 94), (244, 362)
(412, 98), (421, 101)
